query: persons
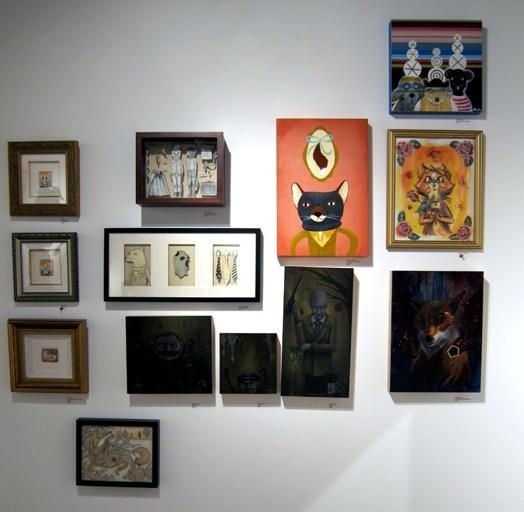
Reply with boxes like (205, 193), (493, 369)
(288, 288), (337, 395)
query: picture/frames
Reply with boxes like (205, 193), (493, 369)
(8, 141), (80, 216)
(387, 129), (483, 248)
(104, 228), (261, 302)
(136, 132), (225, 207)
(76, 418), (160, 488)
(8, 319), (89, 394)
(12, 233), (78, 302)
